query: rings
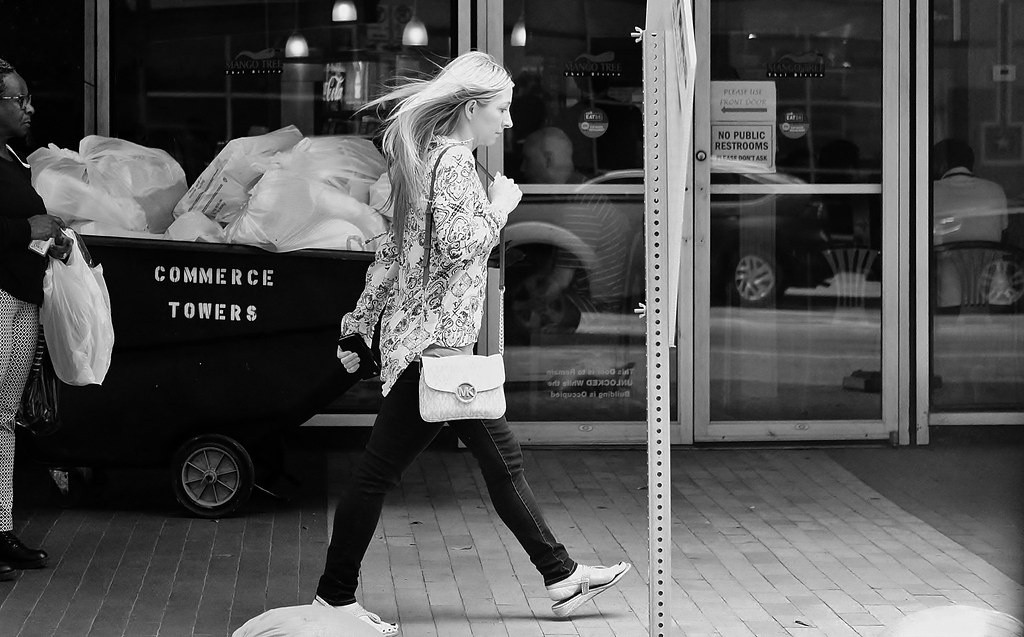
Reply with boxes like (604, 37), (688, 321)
(62, 253), (67, 259)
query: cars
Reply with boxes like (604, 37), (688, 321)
(500, 165), (835, 347)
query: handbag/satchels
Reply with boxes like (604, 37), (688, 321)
(419, 354), (507, 423)
(42, 223), (115, 387)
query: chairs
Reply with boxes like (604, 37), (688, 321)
(810, 241), (882, 378)
(929, 240), (1024, 391)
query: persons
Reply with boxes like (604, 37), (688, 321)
(921, 140), (1014, 404)
(312, 51), (633, 637)
(0, 59), (73, 578)
(516, 127), (634, 380)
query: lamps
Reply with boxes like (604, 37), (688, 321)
(331, 0), (357, 21)
(401, 0), (430, 47)
(510, 5), (527, 47)
(285, 12), (309, 58)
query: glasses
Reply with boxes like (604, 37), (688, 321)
(2, 95), (32, 109)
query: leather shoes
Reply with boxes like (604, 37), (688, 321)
(0, 532), (48, 569)
(0, 560), (16, 581)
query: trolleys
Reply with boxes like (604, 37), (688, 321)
(44, 228), (400, 521)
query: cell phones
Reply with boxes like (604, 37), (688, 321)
(338, 333), (380, 380)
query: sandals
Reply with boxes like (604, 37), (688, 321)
(545, 562), (631, 618)
(311, 595), (399, 637)
